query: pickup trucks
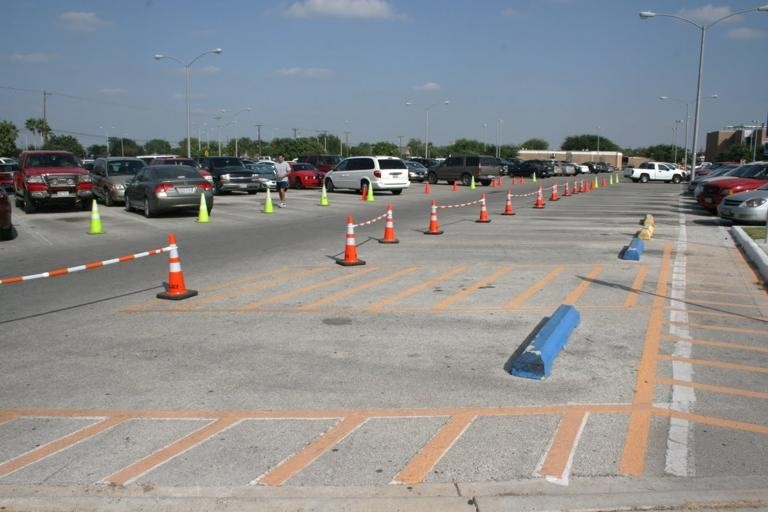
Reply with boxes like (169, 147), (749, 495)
(623, 162), (683, 185)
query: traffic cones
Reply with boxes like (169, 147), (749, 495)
(474, 193), (492, 223)
(365, 182), (375, 202)
(422, 172), (537, 192)
(195, 192), (213, 224)
(334, 217), (367, 268)
(422, 199), (444, 236)
(377, 204), (400, 244)
(152, 233), (200, 302)
(532, 174), (618, 208)
(500, 189), (515, 216)
(86, 199), (107, 234)
(260, 187), (276, 214)
(316, 183), (331, 207)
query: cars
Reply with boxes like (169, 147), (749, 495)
(0, 157), (18, 193)
(714, 182), (768, 224)
(688, 162), (740, 201)
(286, 161), (326, 189)
(696, 162), (768, 213)
(123, 163), (214, 218)
(660, 160), (737, 181)
(245, 163), (290, 191)
(0, 184), (12, 240)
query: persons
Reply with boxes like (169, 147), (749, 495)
(274, 153), (291, 208)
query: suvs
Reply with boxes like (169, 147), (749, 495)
(149, 158), (213, 190)
(426, 154), (502, 186)
(12, 149), (94, 215)
(199, 158), (261, 195)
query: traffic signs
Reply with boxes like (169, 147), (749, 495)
(358, 184), (367, 200)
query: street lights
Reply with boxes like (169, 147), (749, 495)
(404, 100), (449, 157)
(670, 119), (685, 163)
(638, 5), (768, 185)
(152, 47), (222, 157)
(749, 120), (766, 161)
(483, 122), (488, 152)
(221, 107), (252, 156)
(497, 120), (504, 158)
(196, 115), (220, 151)
(656, 95), (721, 169)
(596, 124), (601, 159)
(95, 125), (116, 154)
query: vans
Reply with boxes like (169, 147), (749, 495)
(91, 156), (147, 207)
(323, 157), (411, 195)
